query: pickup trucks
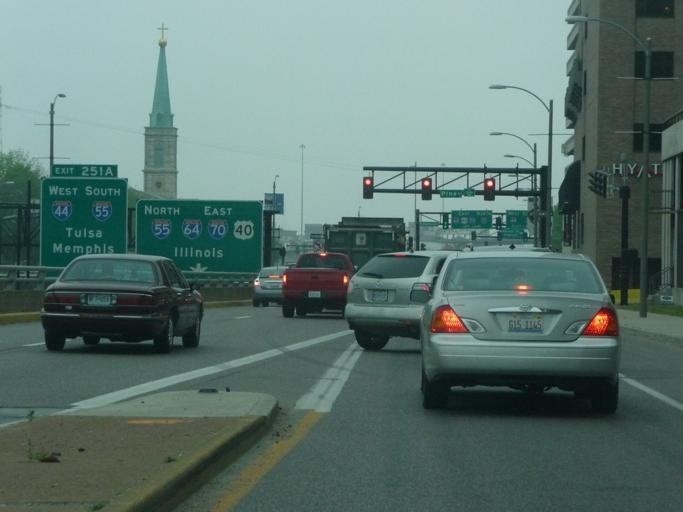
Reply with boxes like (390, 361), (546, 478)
(281, 253), (354, 318)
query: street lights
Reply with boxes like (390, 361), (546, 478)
(489, 131), (537, 189)
(566, 15), (652, 315)
(490, 84), (554, 246)
(48, 94), (66, 175)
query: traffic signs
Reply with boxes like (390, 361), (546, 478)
(440, 190), (462, 198)
(38, 164), (127, 277)
(135, 199), (264, 276)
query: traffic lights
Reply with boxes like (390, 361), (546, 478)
(484, 178), (495, 201)
(443, 213), (449, 229)
(453, 211), (492, 229)
(421, 178), (433, 201)
(496, 217), (501, 229)
(362, 177), (374, 199)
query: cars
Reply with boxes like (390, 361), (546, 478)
(41, 253), (204, 353)
(252, 266), (291, 307)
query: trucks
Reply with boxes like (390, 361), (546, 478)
(323, 216), (406, 272)
